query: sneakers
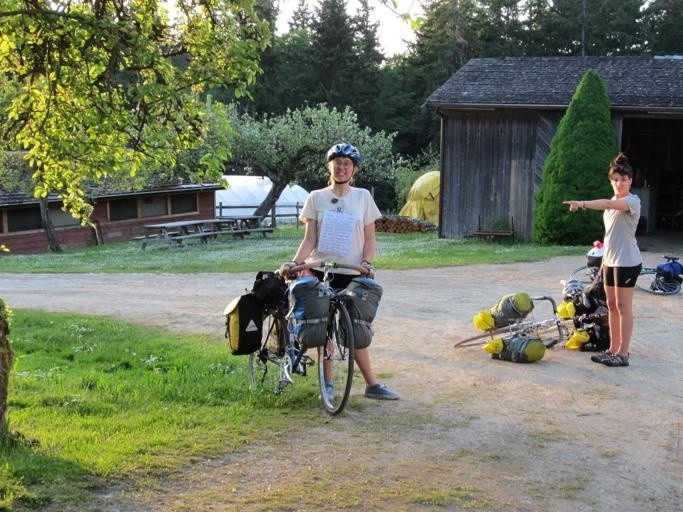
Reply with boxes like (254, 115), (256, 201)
(317, 378), (334, 405)
(590, 349), (630, 368)
(363, 383), (400, 400)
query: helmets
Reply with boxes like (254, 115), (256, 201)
(325, 143), (362, 164)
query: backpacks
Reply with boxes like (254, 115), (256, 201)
(221, 271), (287, 360)
(656, 261), (682, 279)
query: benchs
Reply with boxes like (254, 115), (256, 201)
(133, 214), (276, 251)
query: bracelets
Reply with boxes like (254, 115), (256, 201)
(291, 260), (299, 266)
(360, 259), (369, 267)
(582, 200), (586, 211)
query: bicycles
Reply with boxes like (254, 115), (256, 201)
(454, 255), (683, 351)
(248, 262), (369, 416)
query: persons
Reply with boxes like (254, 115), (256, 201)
(272, 139), (399, 402)
(559, 150), (643, 367)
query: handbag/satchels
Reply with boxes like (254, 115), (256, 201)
(583, 271), (607, 310)
